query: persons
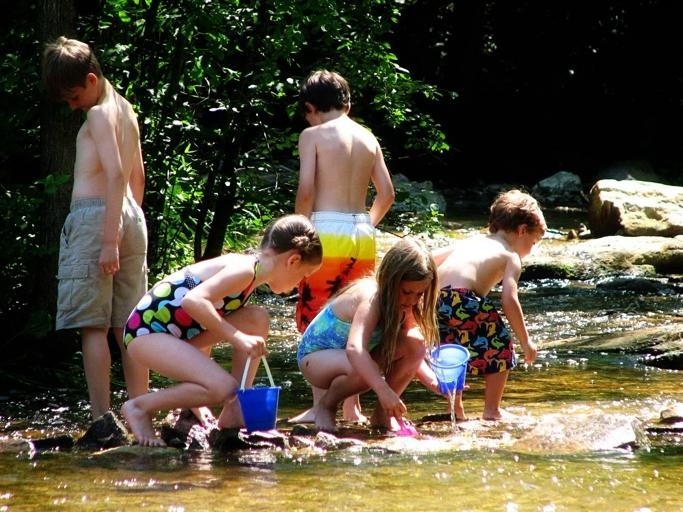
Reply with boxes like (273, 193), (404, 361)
(428, 189), (547, 426)
(284, 71), (398, 421)
(41, 37), (150, 447)
(297, 243), (470, 435)
(121, 211), (322, 446)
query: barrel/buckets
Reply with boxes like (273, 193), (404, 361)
(429, 343), (470, 392)
(233, 353), (282, 433)
